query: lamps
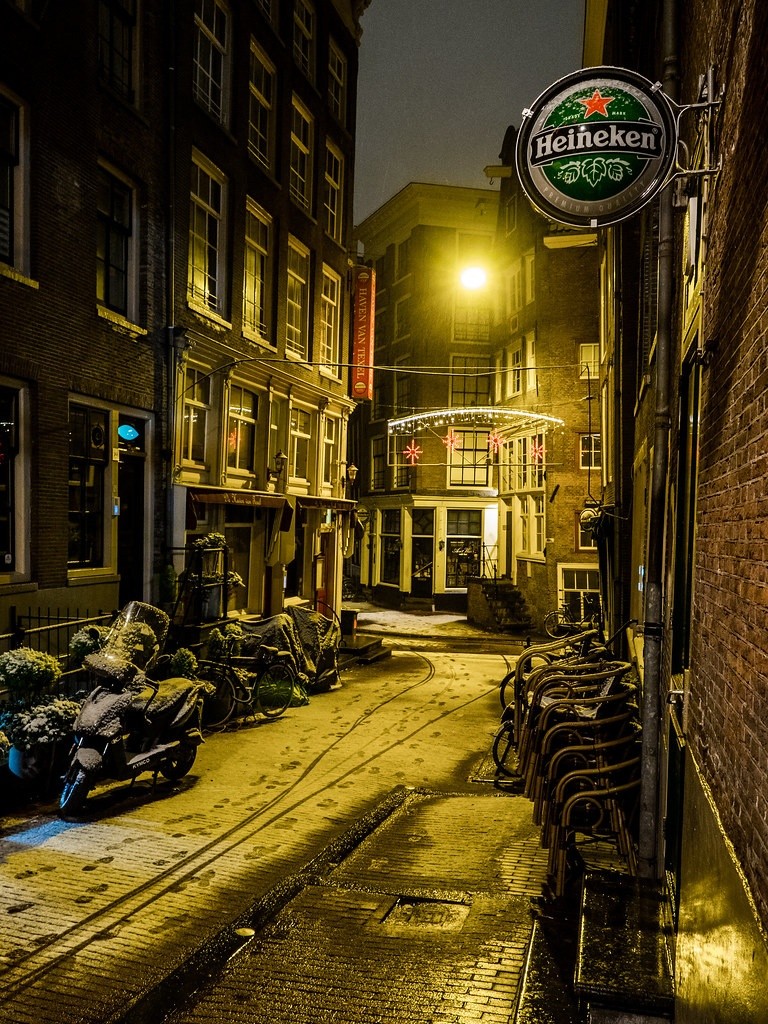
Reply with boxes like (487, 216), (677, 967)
(267, 448), (288, 481)
(342, 462), (359, 488)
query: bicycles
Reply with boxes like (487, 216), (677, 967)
(543, 595), (604, 641)
(192, 628), (298, 726)
(498, 667), (538, 714)
(492, 717), (523, 776)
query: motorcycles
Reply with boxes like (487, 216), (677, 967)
(57, 600), (215, 818)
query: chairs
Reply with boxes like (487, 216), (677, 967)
(513, 630), (644, 896)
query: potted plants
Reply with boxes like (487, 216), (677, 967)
(0, 624), (111, 787)
(171, 623), (251, 723)
(185, 531), (243, 624)
